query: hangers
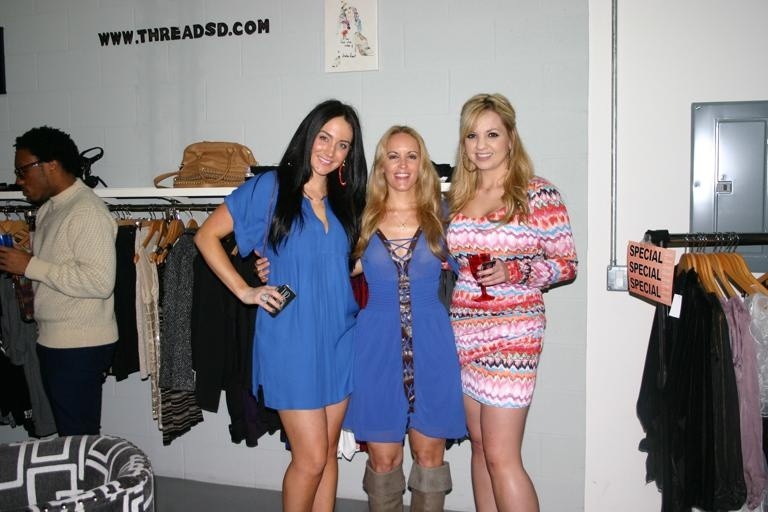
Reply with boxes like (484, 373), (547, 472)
(0, 203), (240, 263)
(674, 233), (768, 322)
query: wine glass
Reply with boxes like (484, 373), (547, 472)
(466, 253), (496, 303)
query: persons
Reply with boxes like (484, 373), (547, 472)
(0, 123), (120, 435)
(252, 125), (469, 511)
(191, 97), (366, 511)
(445, 89), (579, 512)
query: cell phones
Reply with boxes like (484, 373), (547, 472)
(264, 285), (296, 319)
(480, 261), (497, 278)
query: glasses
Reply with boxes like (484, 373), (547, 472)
(15, 159), (44, 179)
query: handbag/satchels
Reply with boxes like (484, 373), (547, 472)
(153, 141), (258, 189)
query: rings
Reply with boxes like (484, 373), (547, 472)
(261, 294), (269, 302)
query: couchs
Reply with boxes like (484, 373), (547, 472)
(0, 434), (155, 512)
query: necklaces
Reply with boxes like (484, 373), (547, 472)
(387, 201), (418, 232)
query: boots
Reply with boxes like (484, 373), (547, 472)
(362, 459), (406, 511)
(407, 460), (452, 512)
